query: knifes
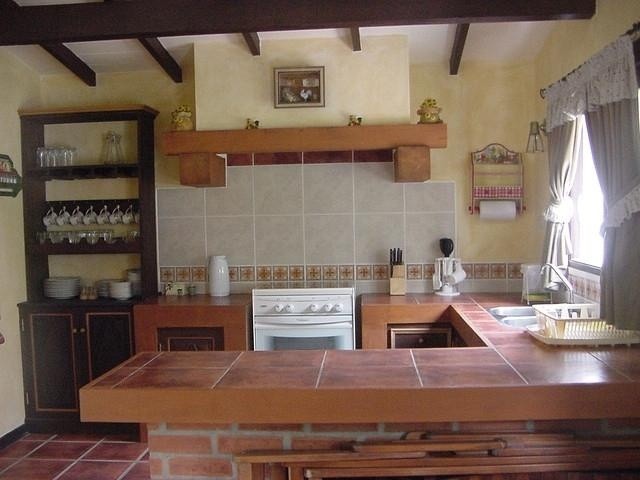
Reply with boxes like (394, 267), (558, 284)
(389, 246), (403, 276)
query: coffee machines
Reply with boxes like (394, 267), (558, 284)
(431, 237), (466, 297)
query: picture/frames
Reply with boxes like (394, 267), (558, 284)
(273, 67), (326, 109)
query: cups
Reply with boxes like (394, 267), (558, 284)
(41, 230), (138, 243)
(38, 206), (137, 225)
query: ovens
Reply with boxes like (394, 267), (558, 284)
(252, 289), (355, 350)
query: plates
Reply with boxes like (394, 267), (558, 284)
(47, 268), (139, 302)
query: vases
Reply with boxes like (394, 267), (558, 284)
(171, 113), (194, 131)
(417, 108), (440, 123)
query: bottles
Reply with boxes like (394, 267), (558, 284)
(35, 144), (76, 167)
(174, 284), (197, 296)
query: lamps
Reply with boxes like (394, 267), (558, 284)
(526, 118), (548, 155)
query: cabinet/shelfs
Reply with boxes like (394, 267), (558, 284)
(17, 102), (159, 297)
(387, 323), (454, 349)
(17, 300), (137, 436)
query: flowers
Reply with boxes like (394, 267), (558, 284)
(172, 103), (192, 113)
(419, 98), (437, 109)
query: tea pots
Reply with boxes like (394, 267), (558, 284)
(100, 130), (126, 165)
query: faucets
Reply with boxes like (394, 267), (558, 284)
(539, 263), (575, 304)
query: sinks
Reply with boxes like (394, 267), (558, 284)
(491, 307), (538, 316)
(504, 317), (538, 327)
